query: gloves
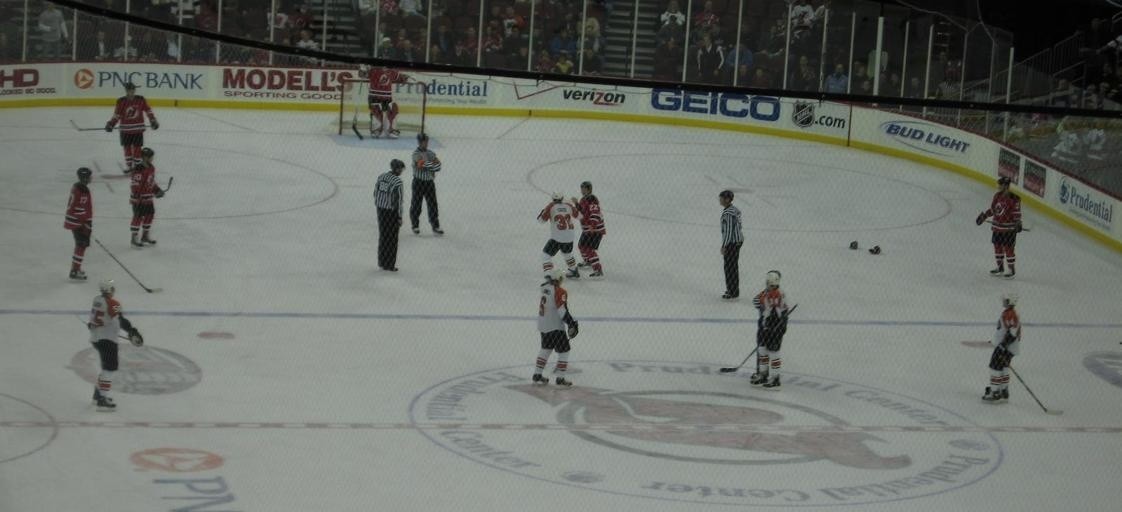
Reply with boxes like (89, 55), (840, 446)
(976, 211), (986, 225)
(155, 189), (165, 197)
(83, 225), (90, 236)
(1014, 221), (1022, 232)
(104, 121), (112, 132)
(129, 328), (144, 345)
(567, 321), (579, 338)
(150, 120), (159, 130)
(135, 195), (144, 205)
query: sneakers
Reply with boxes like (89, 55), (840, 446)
(98, 397), (115, 407)
(372, 130), (381, 137)
(93, 389), (112, 401)
(131, 235), (145, 247)
(990, 268), (1004, 273)
(763, 378), (780, 387)
(750, 376), (768, 385)
(378, 263), (384, 267)
(532, 374), (549, 382)
(722, 294), (738, 298)
(383, 266), (398, 271)
(556, 377), (572, 385)
(589, 272), (604, 277)
(387, 129), (399, 135)
(1004, 271), (1015, 277)
(540, 282), (549, 287)
(725, 291), (729, 294)
(577, 262), (592, 267)
(565, 270), (580, 278)
(78, 270), (85, 274)
(69, 271), (86, 279)
(123, 165), (132, 173)
(982, 392), (1001, 400)
(1000, 389), (1008, 399)
(433, 227), (444, 233)
(413, 227), (420, 233)
(141, 236), (156, 243)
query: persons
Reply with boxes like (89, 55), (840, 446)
(719, 190), (744, 301)
(993, 37), (1122, 197)
(751, 270), (788, 391)
(533, 269), (579, 389)
(374, 159), (407, 273)
(982, 291), (1023, 404)
(87, 277), (144, 411)
(105, 82), (160, 174)
(976, 178), (1024, 280)
(63, 167), (93, 282)
(411, 133), (443, 236)
(350, 0), (612, 77)
(359, 61), (418, 140)
(1, 0), (328, 69)
(655, 0), (992, 131)
(130, 148), (165, 249)
(537, 191), (582, 280)
(568, 181), (607, 279)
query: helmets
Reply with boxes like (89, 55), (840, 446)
(1003, 292), (1019, 306)
(553, 191), (563, 201)
(997, 177), (1011, 185)
(390, 159), (405, 171)
(77, 167), (92, 180)
(141, 147), (153, 160)
(99, 279), (115, 294)
(581, 182), (592, 194)
(767, 271), (779, 286)
(545, 268), (563, 282)
(417, 132), (430, 142)
(719, 190), (734, 200)
(125, 83), (135, 91)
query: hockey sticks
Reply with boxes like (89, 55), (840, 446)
(352, 76), (364, 140)
(719, 304), (798, 373)
(94, 239), (164, 294)
(142, 177), (173, 200)
(70, 120), (152, 131)
(1007, 365), (1063, 415)
